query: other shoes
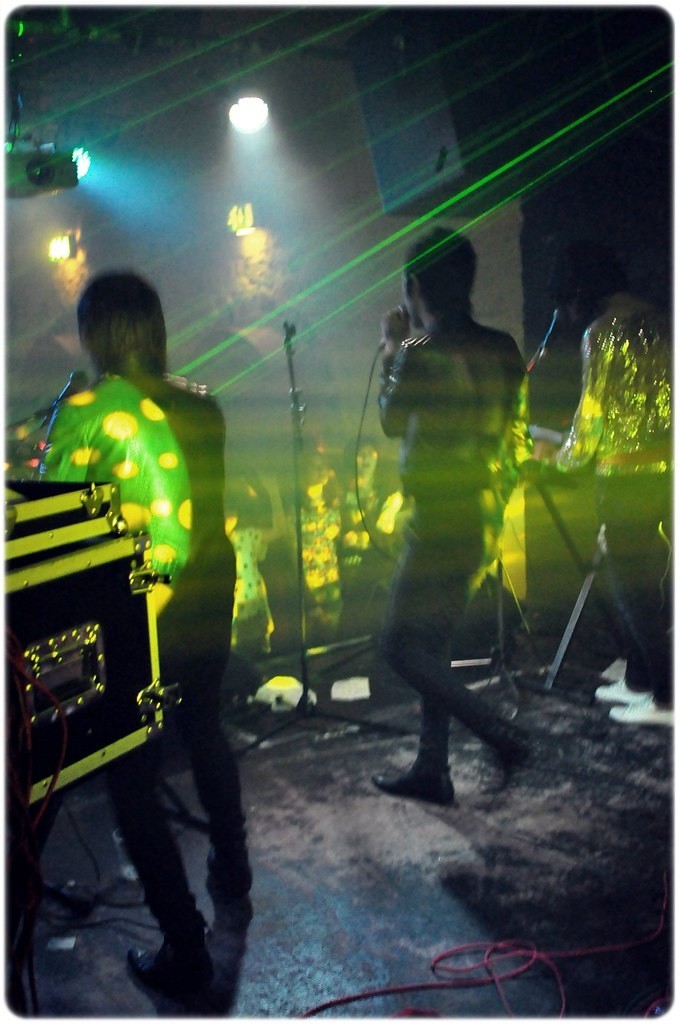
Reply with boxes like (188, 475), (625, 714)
(609, 696), (672, 726)
(478, 729), (531, 793)
(595, 679), (651, 704)
(207, 844), (252, 895)
(372, 763), (456, 804)
(126, 943), (212, 995)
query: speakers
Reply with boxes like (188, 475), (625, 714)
(345, 8), (524, 220)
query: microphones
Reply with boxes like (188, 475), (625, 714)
(41, 370), (88, 429)
(379, 305), (409, 352)
(538, 307), (564, 361)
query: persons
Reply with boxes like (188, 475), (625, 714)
(371, 223), (553, 806)
(37, 269), (250, 999)
(222, 397), (581, 658)
(545, 245), (674, 724)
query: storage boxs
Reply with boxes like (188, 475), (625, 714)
(6, 475), (180, 809)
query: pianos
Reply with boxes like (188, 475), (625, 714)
(519, 459), (582, 489)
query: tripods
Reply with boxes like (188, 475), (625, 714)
(230, 321), (409, 761)
